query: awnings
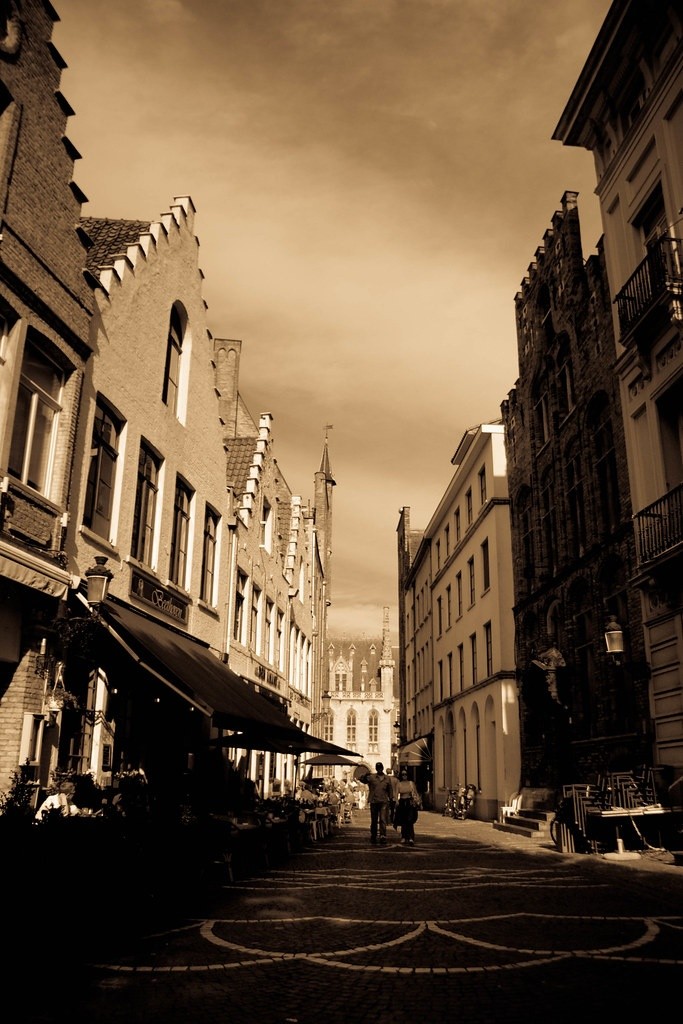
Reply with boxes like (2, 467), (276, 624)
(399, 737), (433, 767)
(76, 589), (310, 739)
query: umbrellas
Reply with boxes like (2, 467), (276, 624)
(208, 718), (364, 800)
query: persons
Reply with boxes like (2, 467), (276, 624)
(265, 778), (367, 838)
(359, 761), (424, 843)
(34, 782), (140, 824)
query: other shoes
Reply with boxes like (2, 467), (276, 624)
(410, 839), (414, 847)
(380, 838), (387, 844)
(400, 838), (405, 843)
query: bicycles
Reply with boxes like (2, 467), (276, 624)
(440, 783), (476, 821)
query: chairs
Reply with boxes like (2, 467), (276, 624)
(209, 796), (355, 883)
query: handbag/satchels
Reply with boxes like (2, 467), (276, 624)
(410, 782), (422, 807)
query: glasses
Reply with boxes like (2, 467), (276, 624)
(402, 773), (407, 776)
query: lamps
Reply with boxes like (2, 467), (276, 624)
(34, 556), (114, 675)
(604, 614), (651, 682)
